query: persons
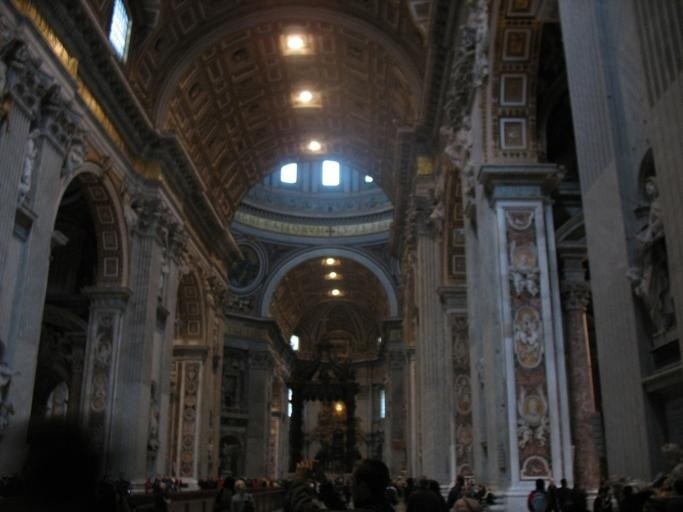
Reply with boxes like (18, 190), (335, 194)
(214, 477), (256, 511)
(631, 176), (674, 327)
(625, 265), (669, 337)
(528, 467), (682, 512)
(2, 416), (172, 510)
(16, 128), (42, 201)
(283, 460), (486, 510)
